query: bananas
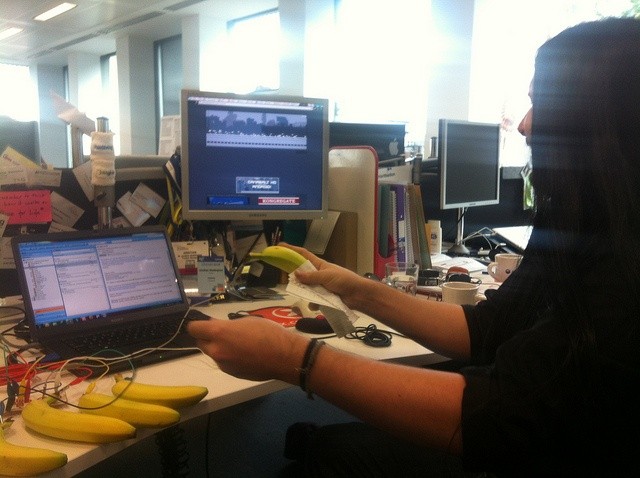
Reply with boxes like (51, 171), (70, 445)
(21, 399), (136, 443)
(248, 246), (306, 274)
(78, 382), (181, 427)
(112, 372), (208, 408)
(0, 425), (68, 477)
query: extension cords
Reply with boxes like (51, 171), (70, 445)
(1, 388), (67, 414)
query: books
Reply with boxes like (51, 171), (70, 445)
(377, 182), (432, 275)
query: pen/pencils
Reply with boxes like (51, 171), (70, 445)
(269, 227), (281, 246)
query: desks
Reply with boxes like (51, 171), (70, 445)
(1, 283), (451, 474)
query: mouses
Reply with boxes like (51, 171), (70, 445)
(291, 300), (323, 317)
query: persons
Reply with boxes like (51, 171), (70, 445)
(187, 17), (640, 478)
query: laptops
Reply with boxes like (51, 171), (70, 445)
(10, 224), (211, 379)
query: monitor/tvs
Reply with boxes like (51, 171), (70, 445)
(329, 121), (406, 167)
(180, 86), (329, 285)
(437, 116), (502, 256)
(0, 120), (42, 167)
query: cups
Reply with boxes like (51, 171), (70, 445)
(487, 254), (523, 282)
(385, 261), (420, 296)
(441, 281), (487, 306)
